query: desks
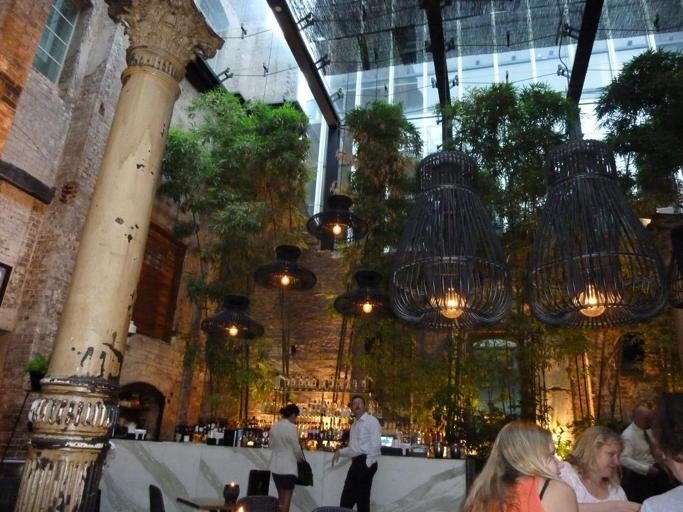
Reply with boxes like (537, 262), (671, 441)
(97, 435), (469, 511)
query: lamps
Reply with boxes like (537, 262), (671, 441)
(254, 241), (315, 289)
(388, 1), (516, 331)
(334, 265), (394, 322)
(202, 289), (267, 343)
(305, 48), (372, 244)
(525, 2), (671, 329)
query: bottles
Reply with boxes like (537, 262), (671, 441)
(281, 374), (366, 392)
(261, 399), (353, 417)
(450, 443), (461, 459)
(258, 421), (349, 440)
(433, 441), (445, 459)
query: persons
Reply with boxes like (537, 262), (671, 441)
(463, 421), (579, 512)
(268, 404), (303, 512)
(640, 391), (683, 512)
(618, 405), (669, 502)
(559, 425), (641, 512)
(331, 395), (382, 512)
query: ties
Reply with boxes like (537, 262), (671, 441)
(643, 430), (654, 458)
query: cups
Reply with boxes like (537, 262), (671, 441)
(213, 427), (224, 447)
(192, 433), (201, 442)
(207, 427), (216, 445)
(221, 482), (240, 506)
(174, 432), (190, 442)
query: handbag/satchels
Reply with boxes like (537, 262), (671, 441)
(294, 458), (313, 486)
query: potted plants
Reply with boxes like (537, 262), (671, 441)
(24, 352), (48, 391)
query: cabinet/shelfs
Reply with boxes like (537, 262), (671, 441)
(270, 380), (383, 438)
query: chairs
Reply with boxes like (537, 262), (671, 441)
(235, 495), (278, 512)
(309, 504), (356, 512)
(146, 482), (168, 511)
